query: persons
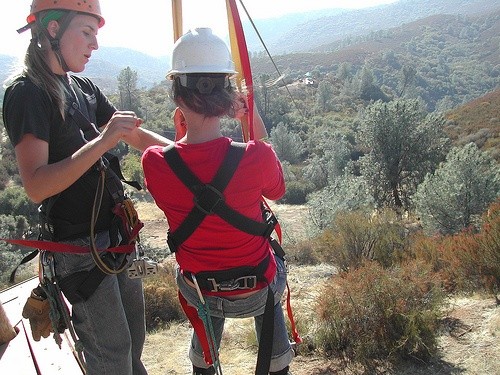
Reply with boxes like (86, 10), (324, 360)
(2, 0), (174, 375)
(142, 26), (295, 375)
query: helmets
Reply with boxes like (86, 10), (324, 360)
(26, 0), (105, 29)
(165, 26), (239, 81)
